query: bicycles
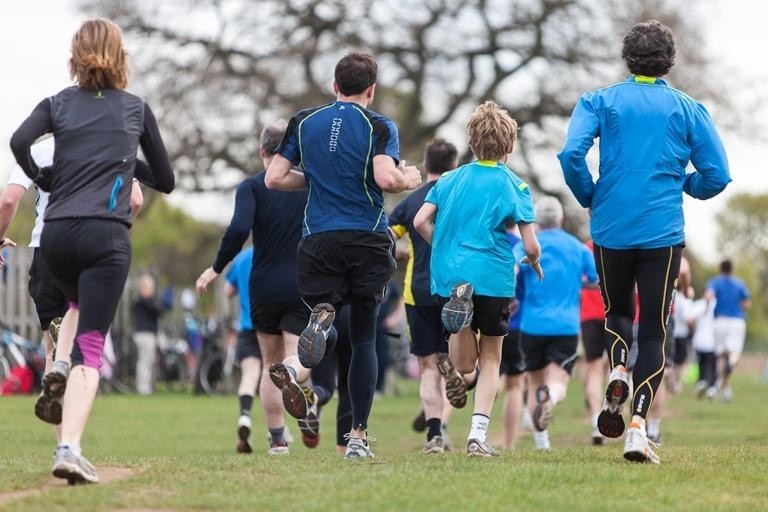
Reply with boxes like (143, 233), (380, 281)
(100, 312), (245, 399)
(0, 317), (56, 393)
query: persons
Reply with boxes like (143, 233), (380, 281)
(196, 122), (310, 457)
(1, 135), (144, 448)
(556, 19), (732, 463)
(264, 52), (423, 465)
(8, 17), (176, 484)
(413, 100), (545, 461)
(389, 138), (462, 453)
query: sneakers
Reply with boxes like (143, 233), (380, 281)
(50, 444), (99, 484)
(48, 315), (63, 362)
(268, 362), (308, 420)
(33, 359), (73, 425)
(414, 407), (663, 466)
(440, 280), (474, 335)
(532, 383), (554, 433)
(236, 415), (254, 454)
(267, 386), (320, 458)
(436, 353), (468, 409)
(595, 368), (631, 442)
(296, 302), (335, 369)
(345, 436), (376, 459)
